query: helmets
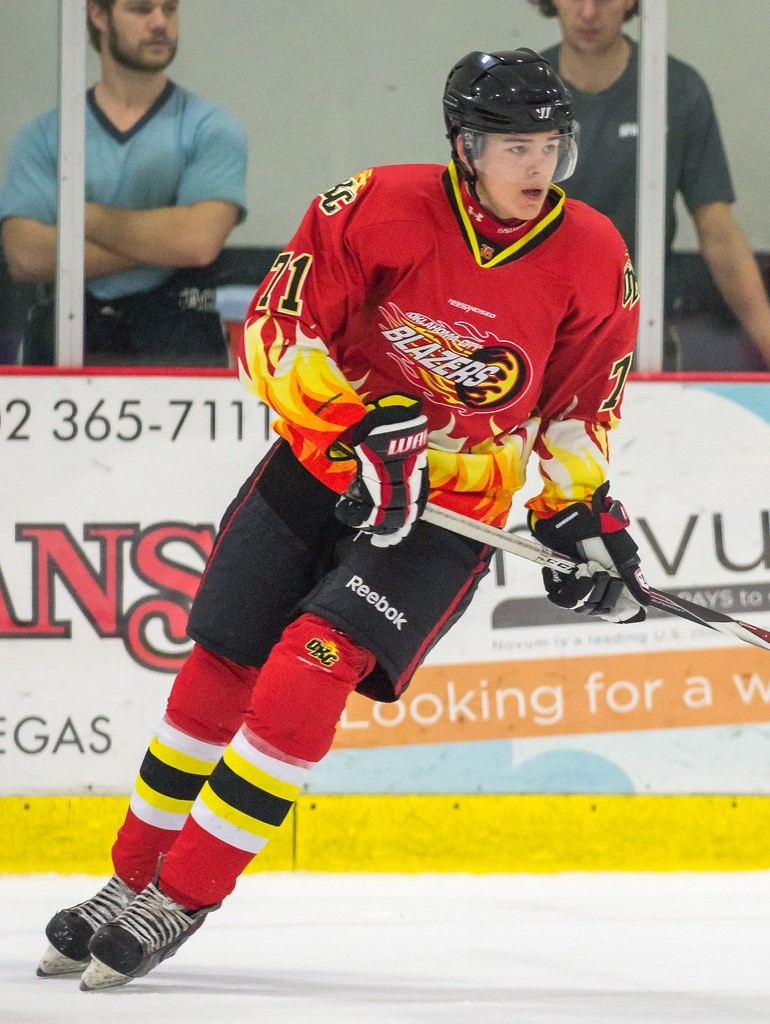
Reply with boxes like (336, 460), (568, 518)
(443, 48), (575, 155)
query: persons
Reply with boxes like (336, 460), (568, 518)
(36, 47), (652, 991)
(0, 0), (249, 368)
(524, 0), (770, 372)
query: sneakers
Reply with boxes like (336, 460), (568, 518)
(78, 854), (223, 992)
(35, 873), (137, 977)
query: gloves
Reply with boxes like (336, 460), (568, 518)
(526, 479), (651, 624)
(334, 391), (428, 534)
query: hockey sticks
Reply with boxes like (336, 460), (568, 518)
(348, 476), (770, 652)
